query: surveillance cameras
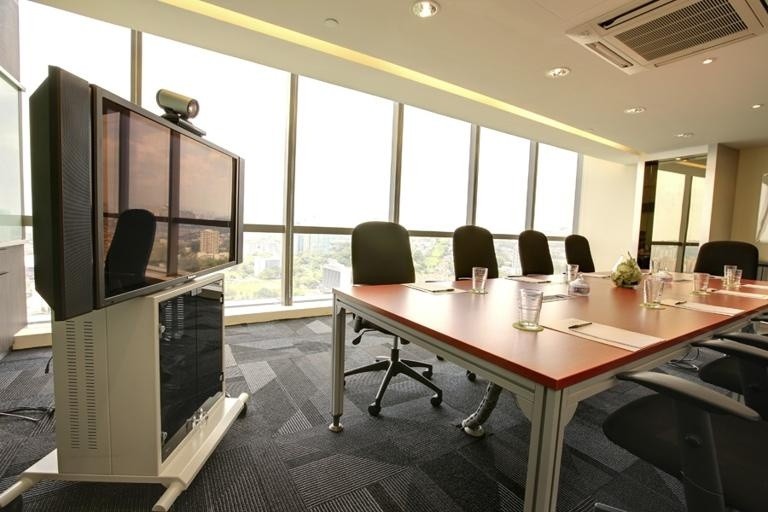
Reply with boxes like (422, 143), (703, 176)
(156, 89), (197, 118)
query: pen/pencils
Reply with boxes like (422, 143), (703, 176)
(709, 276), (715, 278)
(432, 289), (454, 292)
(538, 281), (550, 284)
(569, 323), (592, 329)
(711, 289), (719, 291)
(675, 301), (687, 304)
(740, 283), (746, 285)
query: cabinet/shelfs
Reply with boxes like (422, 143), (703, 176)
(0, 273), (248, 512)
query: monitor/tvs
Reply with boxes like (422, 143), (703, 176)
(93, 88), (240, 307)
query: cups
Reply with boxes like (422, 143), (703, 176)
(644, 279), (663, 308)
(693, 272), (710, 294)
(652, 258), (661, 274)
(519, 289), (544, 329)
(566, 264), (579, 284)
(472, 267), (488, 294)
(724, 265), (738, 286)
(727, 269), (743, 288)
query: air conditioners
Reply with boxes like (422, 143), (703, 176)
(566, 0), (768, 75)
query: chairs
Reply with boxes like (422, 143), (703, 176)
(453, 224), (498, 280)
(564, 234), (595, 273)
(693, 241), (758, 281)
(518, 230), (555, 276)
(595, 339), (767, 512)
(691, 313), (768, 396)
(342, 222), (443, 415)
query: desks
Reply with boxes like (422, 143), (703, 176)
(329, 271), (768, 512)
